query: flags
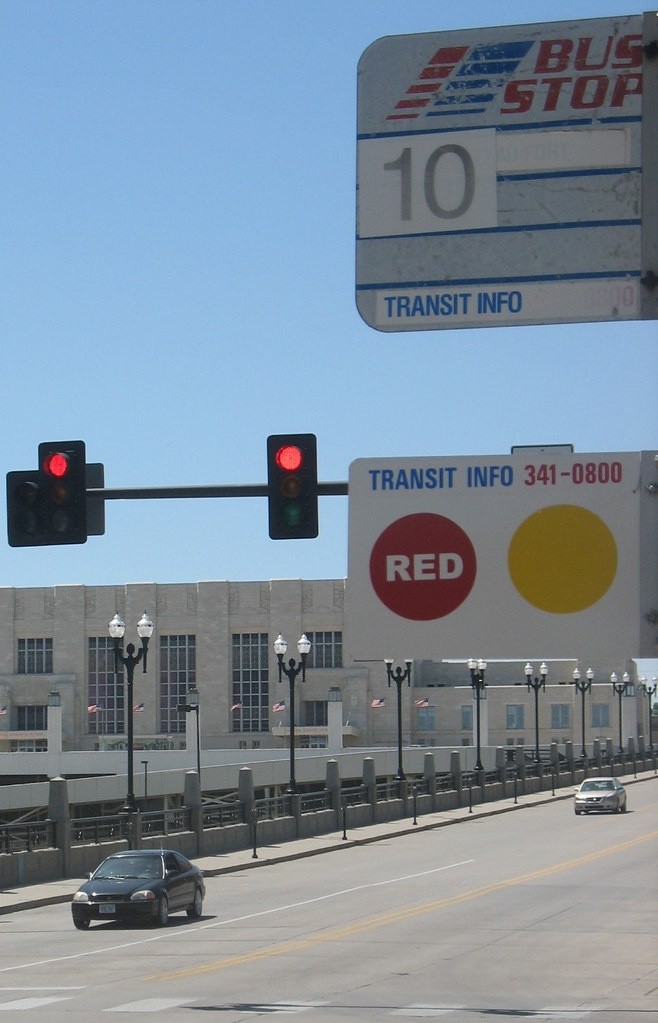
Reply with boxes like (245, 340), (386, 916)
(415, 698), (428, 708)
(231, 702), (241, 711)
(0, 706), (6, 715)
(134, 703), (144, 712)
(272, 701), (285, 712)
(371, 698), (385, 707)
(88, 704), (102, 713)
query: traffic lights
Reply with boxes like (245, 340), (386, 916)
(267, 433), (319, 540)
(5, 440), (86, 546)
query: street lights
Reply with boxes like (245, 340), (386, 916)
(640, 675), (658, 750)
(273, 631), (312, 794)
(176, 704), (201, 783)
(141, 760), (149, 813)
(107, 609), (154, 813)
(467, 658), (487, 771)
(385, 660), (414, 781)
(610, 671), (631, 753)
(573, 665), (594, 758)
(523, 661), (549, 763)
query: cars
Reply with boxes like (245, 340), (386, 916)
(71, 849), (206, 930)
(574, 777), (627, 815)
